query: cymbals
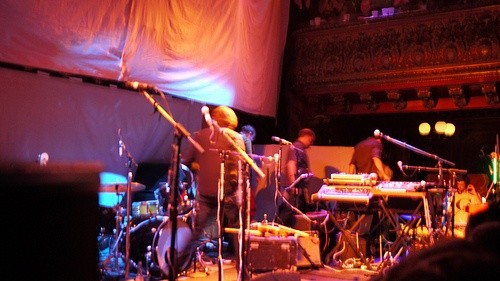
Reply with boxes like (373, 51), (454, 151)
(98, 183), (145, 192)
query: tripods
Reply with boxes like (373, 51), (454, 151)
(99, 185), (147, 274)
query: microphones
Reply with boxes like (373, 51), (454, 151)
(201, 106), (214, 130)
(397, 160), (403, 171)
(374, 129), (393, 141)
(271, 136), (292, 146)
(274, 153), (279, 162)
(123, 80), (157, 92)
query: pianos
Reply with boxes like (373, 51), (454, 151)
(311, 181), (449, 214)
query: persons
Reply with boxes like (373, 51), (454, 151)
(350, 133), (390, 256)
(279, 128), (315, 227)
(171, 105), (248, 273)
(218, 126), (259, 258)
(446, 174), (482, 227)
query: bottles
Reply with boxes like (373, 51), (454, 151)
(98, 227), (109, 261)
(260, 213), (269, 237)
(110, 229), (122, 263)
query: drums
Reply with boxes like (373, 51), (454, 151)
(121, 216), (194, 276)
(154, 181), (197, 217)
